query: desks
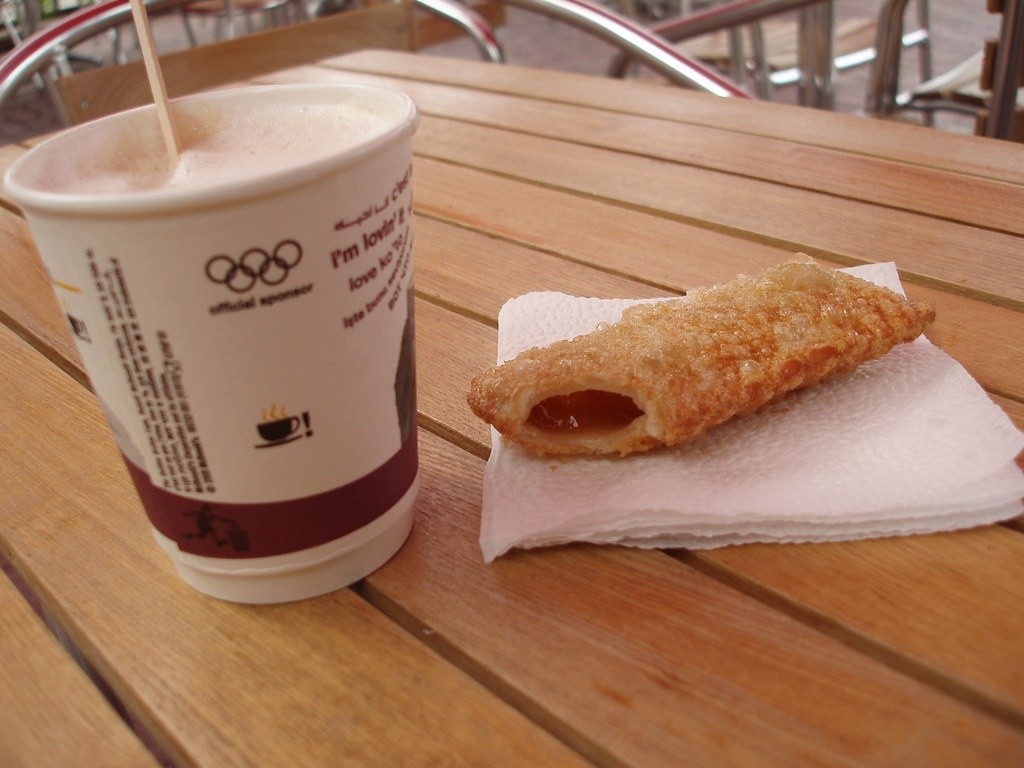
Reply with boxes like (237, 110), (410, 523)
(1, 48), (1024, 767)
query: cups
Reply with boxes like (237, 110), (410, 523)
(4, 85), (420, 604)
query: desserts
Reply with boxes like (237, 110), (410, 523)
(462, 255), (938, 456)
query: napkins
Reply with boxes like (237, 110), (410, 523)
(479, 262), (1023, 564)
